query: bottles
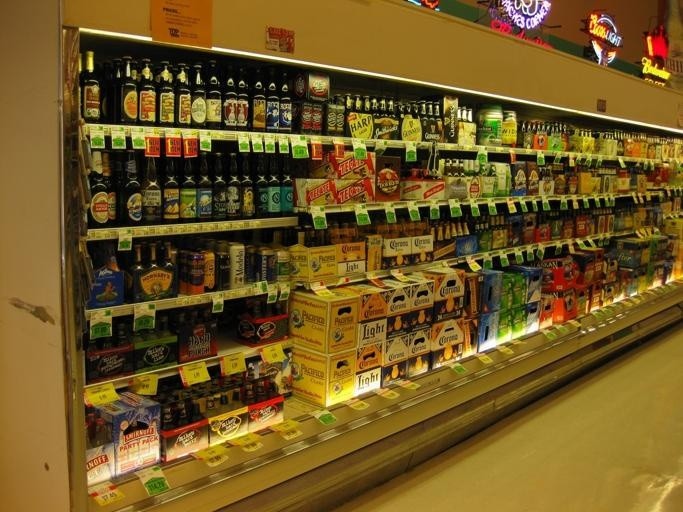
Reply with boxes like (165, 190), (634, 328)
(82, 48), (295, 225)
(439, 156), (512, 199)
(128, 239), (181, 298)
(286, 206), (635, 251)
(302, 76), (475, 144)
(85, 298), (283, 452)
(505, 277), (526, 339)
(520, 120), (681, 157)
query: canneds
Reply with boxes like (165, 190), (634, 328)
(178, 238), (290, 295)
